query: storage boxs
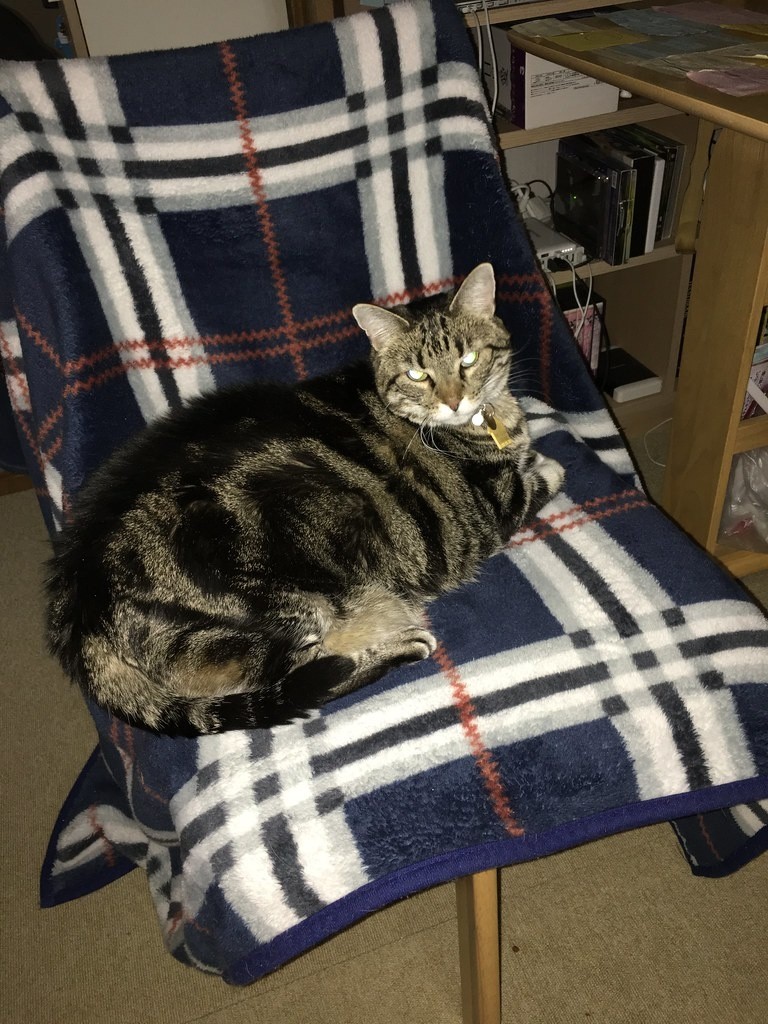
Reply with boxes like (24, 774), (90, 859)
(471, 12), (619, 130)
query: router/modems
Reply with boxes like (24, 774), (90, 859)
(523, 217), (575, 271)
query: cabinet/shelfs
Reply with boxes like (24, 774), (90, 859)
(506, 9), (768, 578)
(332, 0), (722, 420)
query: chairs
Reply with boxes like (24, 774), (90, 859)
(0, 0), (768, 1024)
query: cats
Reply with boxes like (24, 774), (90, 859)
(38, 262), (565, 741)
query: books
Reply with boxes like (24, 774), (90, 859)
(596, 345), (663, 404)
(554, 282), (604, 376)
(739, 339), (768, 421)
